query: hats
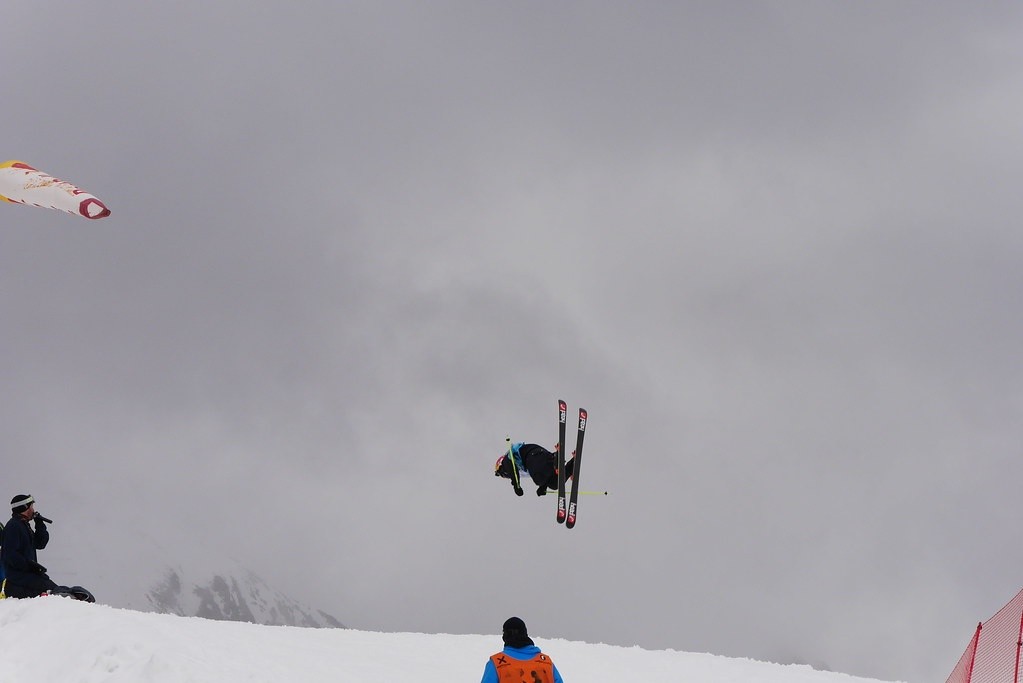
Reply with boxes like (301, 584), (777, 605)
(11, 495), (32, 513)
(503, 617), (527, 640)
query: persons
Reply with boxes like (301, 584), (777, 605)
(481, 617), (564, 683)
(495, 443), (575, 497)
(0, 495), (59, 600)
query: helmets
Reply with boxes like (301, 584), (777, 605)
(494, 456), (507, 478)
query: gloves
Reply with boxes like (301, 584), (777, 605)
(515, 488), (523, 496)
(34, 511), (46, 528)
(537, 486), (547, 497)
(29, 560), (47, 575)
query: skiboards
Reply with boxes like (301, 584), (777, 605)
(556, 399), (587, 531)
